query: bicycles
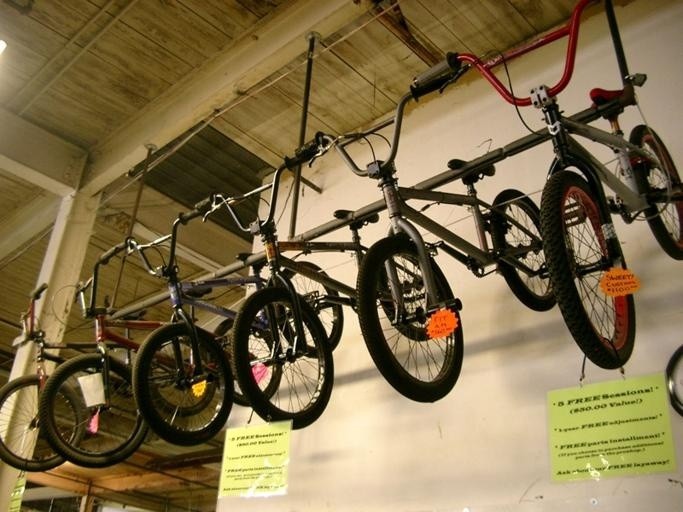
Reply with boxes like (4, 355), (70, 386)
(36, 241), (283, 471)
(0, 282), (217, 473)
(294, 63), (558, 405)
(99, 199), (345, 447)
(412, 0), (682, 370)
(194, 147), (448, 431)
